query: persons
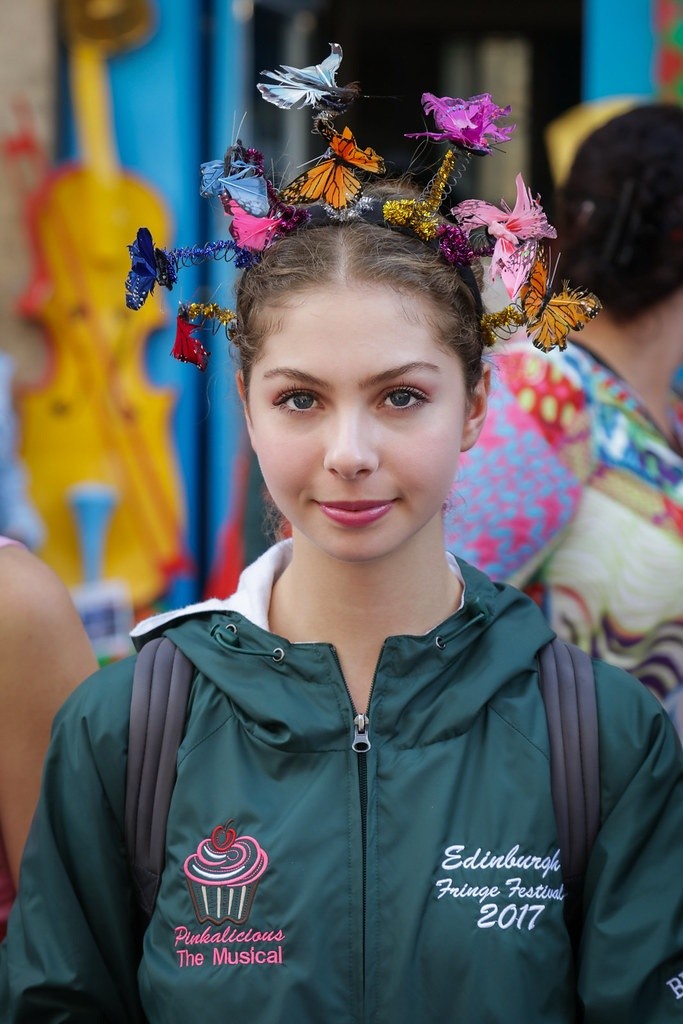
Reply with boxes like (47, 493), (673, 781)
(0, 180), (683, 1024)
(441, 103), (683, 756)
(0, 536), (101, 945)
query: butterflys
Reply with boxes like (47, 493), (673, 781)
(124, 38), (603, 372)
